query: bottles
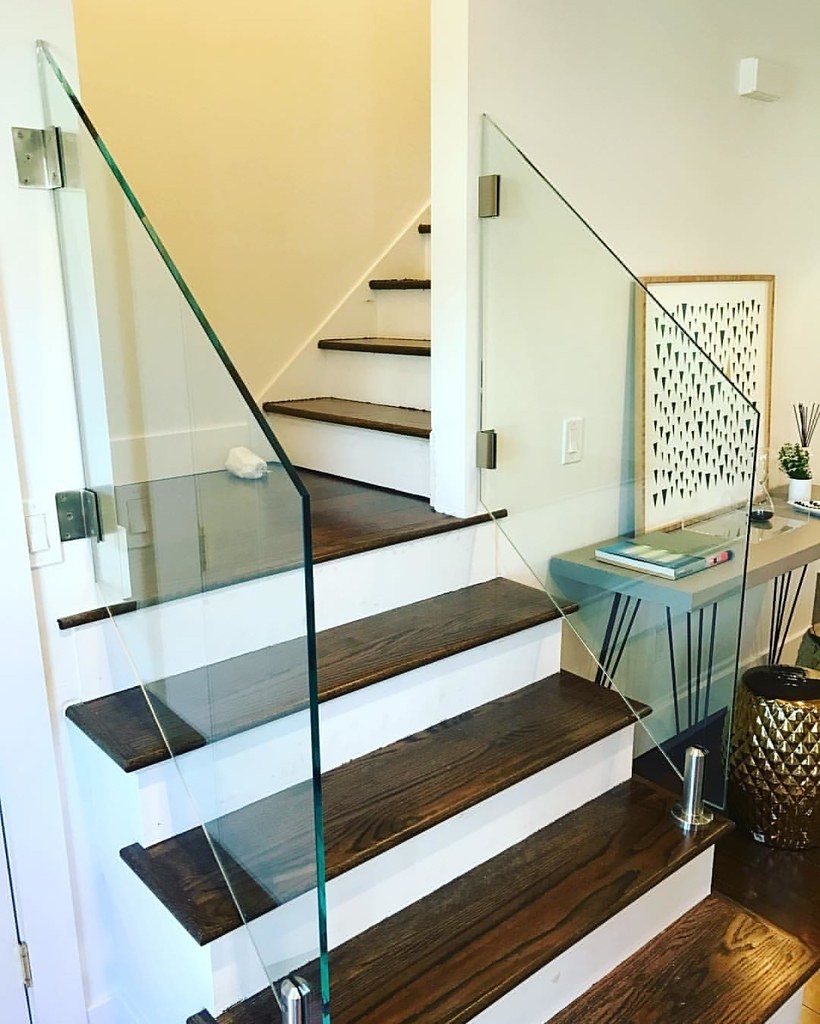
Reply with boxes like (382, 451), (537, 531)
(746, 447), (774, 522)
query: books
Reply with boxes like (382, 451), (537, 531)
(596, 528), (734, 581)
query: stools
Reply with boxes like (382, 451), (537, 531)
(721, 663), (820, 854)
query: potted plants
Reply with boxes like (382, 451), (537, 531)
(777, 442), (814, 504)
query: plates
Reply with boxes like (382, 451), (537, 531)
(787, 500), (820, 516)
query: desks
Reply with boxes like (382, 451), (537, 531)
(550, 484), (817, 767)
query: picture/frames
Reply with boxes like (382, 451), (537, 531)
(634, 274), (776, 541)
